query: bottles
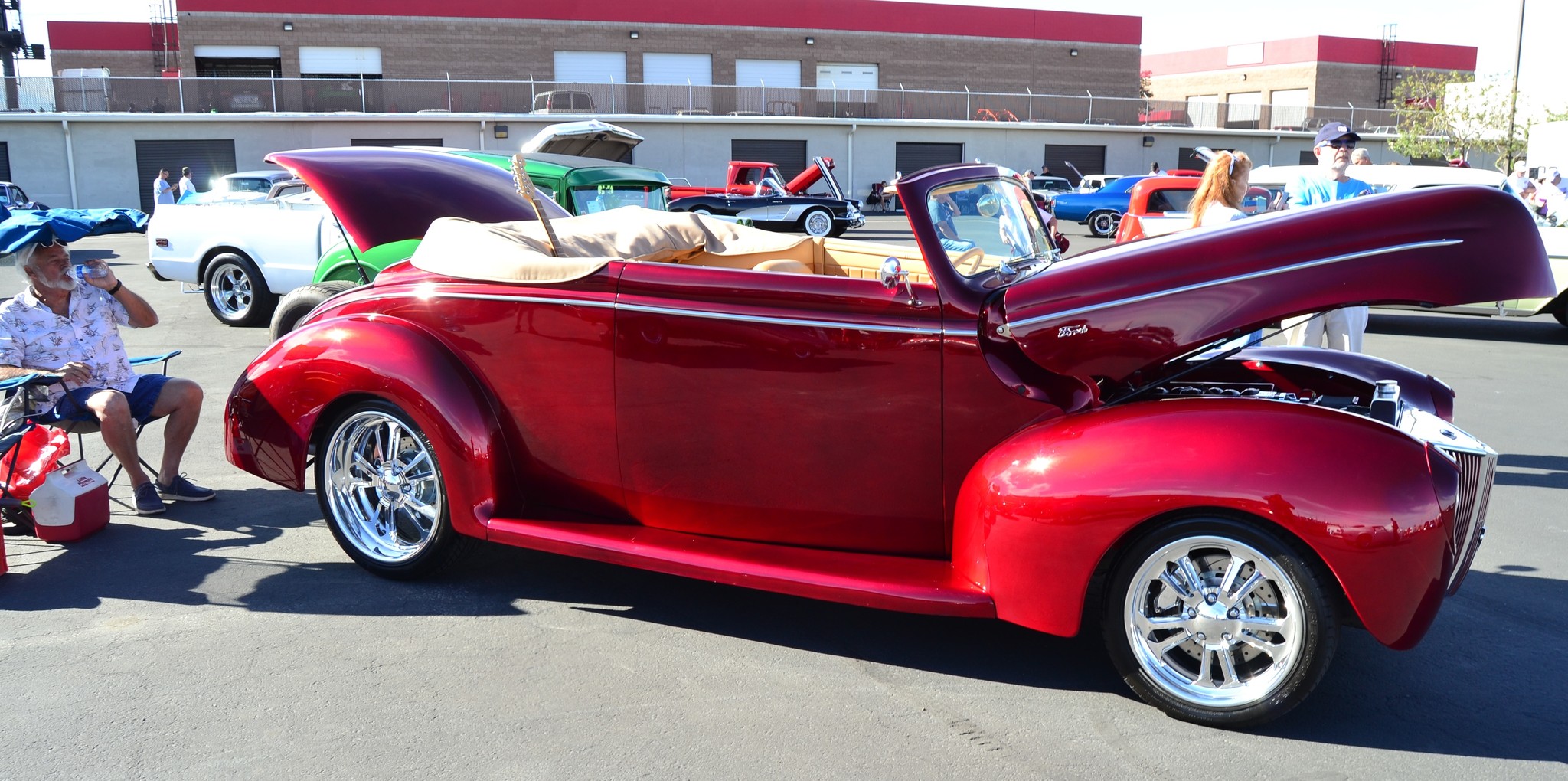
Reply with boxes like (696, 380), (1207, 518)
(65, 262), (108, 280)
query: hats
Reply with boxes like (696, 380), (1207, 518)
(1150, 161), (1158, 171)
(1513, 160), (1527, 173)
(182, 166), (193, 175)
(1545, 168), (1559, 183)
(1314, 121), (1362, 146)
(1024, 169), (1037, 176)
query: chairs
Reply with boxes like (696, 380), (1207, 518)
(754, 260), (813, 274)
(0, 297), (183, 511)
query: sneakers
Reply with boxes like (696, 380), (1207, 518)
(133, 482), (166, 515)
(155, 475), (216, 502)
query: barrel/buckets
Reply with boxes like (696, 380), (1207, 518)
(27, 458), (116, 543)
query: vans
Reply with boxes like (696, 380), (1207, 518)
(529, 90), (597, 113)
(1084, 118), (1117, 125)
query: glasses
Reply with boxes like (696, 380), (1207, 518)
(23, 237), (68, 266)
(163, 173), (168, 176)
(1319, 139), (1356, 149)
(1220, 150), (1240, 176)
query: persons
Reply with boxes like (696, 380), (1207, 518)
(1509, 161), (1567, 227)
(1041, 165), (1052, 176)
(928, 193), (975, 251)
(1149, 161), (1168, 176)
(999, 198), (1057, 256)
(153, 169), (178, 204)
(1188, 150), (1289, 346)
(1, 237), (216, 514)
(1022, 170), (1037, 197)
(129, 97), (217, 113)
(1291, 122), (1369, 352)
(1351, 148), (1372, 165)
(179, 168), (197, 196)
(878, 181), (894, 213)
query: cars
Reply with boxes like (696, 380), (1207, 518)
(727, 111), (764, 116)
(147, 144), (1566, 726)
(0, 182), (50, 214)
(676, 109), (711, 116)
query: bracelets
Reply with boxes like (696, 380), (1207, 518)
(108, 279), (122, 295)
